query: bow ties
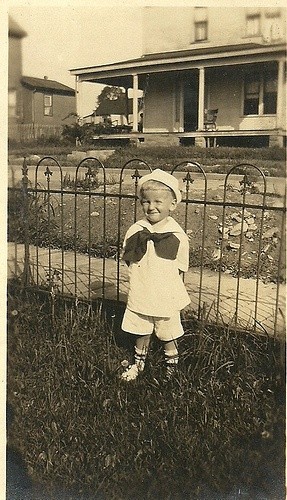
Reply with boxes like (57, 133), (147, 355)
(122, 226), (180, 263)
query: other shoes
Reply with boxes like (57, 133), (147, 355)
(121, 364), (143, 381)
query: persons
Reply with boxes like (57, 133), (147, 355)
(121, 169), (192, 381)
(138, 113), (143, 132)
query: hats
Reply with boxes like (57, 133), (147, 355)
(137, 169), (182, 203)
